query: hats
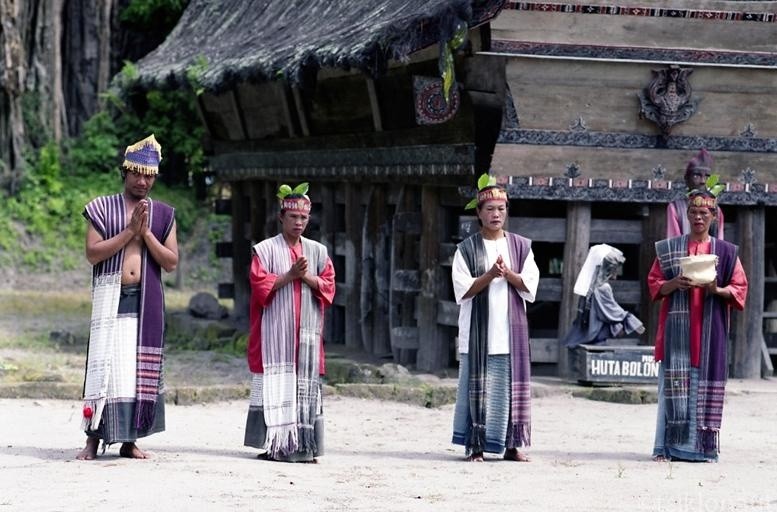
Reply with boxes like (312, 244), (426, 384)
(123, 133), (161, 176)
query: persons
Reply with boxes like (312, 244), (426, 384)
(75, 131), (179, 459)
(653, 147), (725, 243)
(248, 180), (337, 463)
(648, 173), (747, 464)
(451, 172), (543, 462)
(566, 245), (645, 351)
